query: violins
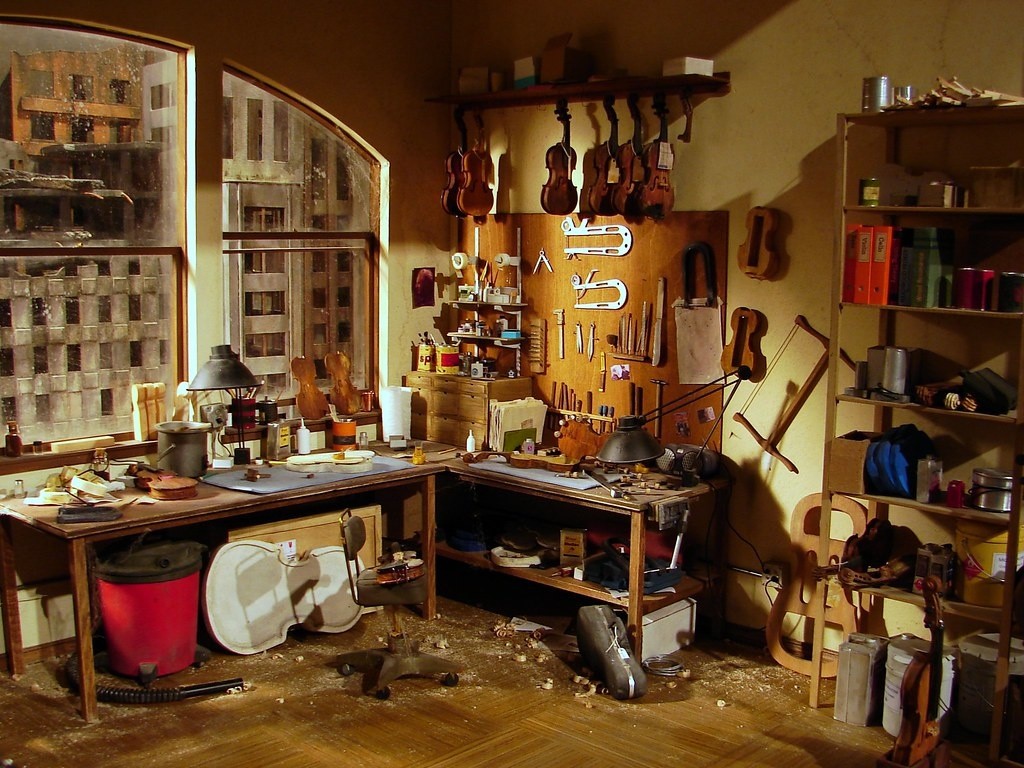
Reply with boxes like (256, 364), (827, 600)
(457, 99), (494, 217)
(440, 99), (469, 215)
(891, 576), (952, 768)
(587, 91), (676, 220)
(540, 95), (578, 215)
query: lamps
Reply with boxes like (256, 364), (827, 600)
(186, 344), (258, 466)
(595, 364), (752, 488)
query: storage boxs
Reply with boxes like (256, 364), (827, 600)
(540, 32), (595, 83)
(642, 597), (697, 664)
(513, 56), (540, 91)
(827, 429), (883, 496)
(456, 65), (493, 96)
(916, 183), (957, 208)
(661, 56), (715, 77)
(574, 552), (606, 581)
(559, 527), (588, 567)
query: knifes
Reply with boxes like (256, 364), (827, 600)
(651, 277), (663, 365)
(598, 351), (606, 392)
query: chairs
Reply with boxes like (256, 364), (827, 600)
(335, 507), (460, 702)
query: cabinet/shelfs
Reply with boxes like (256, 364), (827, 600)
(446, 300), (529, 350)
(405, 370), (532, 452)
(809, 109), (1024, 765)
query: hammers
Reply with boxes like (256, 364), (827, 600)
(650, 378), (669, 438)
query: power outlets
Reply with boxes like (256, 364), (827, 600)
(761, 561), (790, 590)
(199, 402), (229, 433)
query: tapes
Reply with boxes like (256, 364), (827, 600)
(452, 253), (468, 269)
(495, 253), (510, 268)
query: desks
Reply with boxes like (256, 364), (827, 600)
(387, 458), (731, 664)
(0, 448), (446, 722)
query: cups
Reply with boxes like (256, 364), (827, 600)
(258, 399), (278, 425)
(858, 177), (880, 207)
(861, 75), (919, 114)
(231, 397), (256, 430)
(332, 418), (357, 452)
(416, 344), (460, 375)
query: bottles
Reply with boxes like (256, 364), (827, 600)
(358, 432), (369, 450)
(466, 429), (476, 453)
(14, 480), (24, 499)
(92, 448), (111, 483)
(296, 418), (312, 455)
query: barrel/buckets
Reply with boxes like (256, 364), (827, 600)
(154, 421), (210, 478)
(952, 519), (1024, 608)
(832, 631), (954, 738)
(90, 549), (203, 679)
